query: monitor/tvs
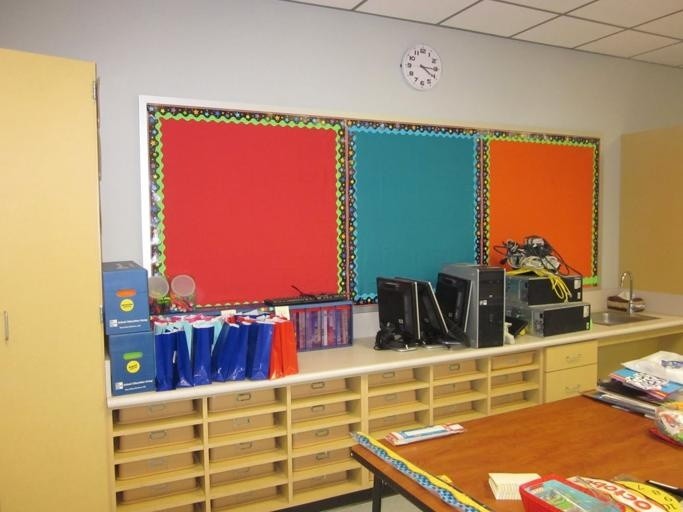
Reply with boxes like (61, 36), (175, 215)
(395, 277), (448, 349)
(376, 276), (421, 353)
(435, 272), (472, 346)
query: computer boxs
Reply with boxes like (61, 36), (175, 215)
(506, 275), (583, 307)
(442, 265), (507, 348)
(506, 301), (592, 337)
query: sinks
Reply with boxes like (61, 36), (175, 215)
(590, 310), (663, 327)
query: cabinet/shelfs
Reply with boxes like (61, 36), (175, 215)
(109, 340), (598, 512)
(0, 50), (116, 511)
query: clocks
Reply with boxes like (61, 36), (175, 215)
(401, 43), (442, 91)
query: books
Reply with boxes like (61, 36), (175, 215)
(580, 347), (682, 422)
(488, 471), (543, 502)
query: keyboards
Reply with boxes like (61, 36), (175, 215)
(264, 293), (345, 307)
(505, 314), (528, 336)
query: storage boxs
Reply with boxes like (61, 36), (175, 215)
(103, 261), (153, 334)
(109, 332), (157, 396)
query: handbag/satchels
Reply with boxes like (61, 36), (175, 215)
(149, 314), (298, 392)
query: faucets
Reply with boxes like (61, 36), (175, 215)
(619, 271), (647, 315)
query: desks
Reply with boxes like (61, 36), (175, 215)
(350, 395), (683, 512)
